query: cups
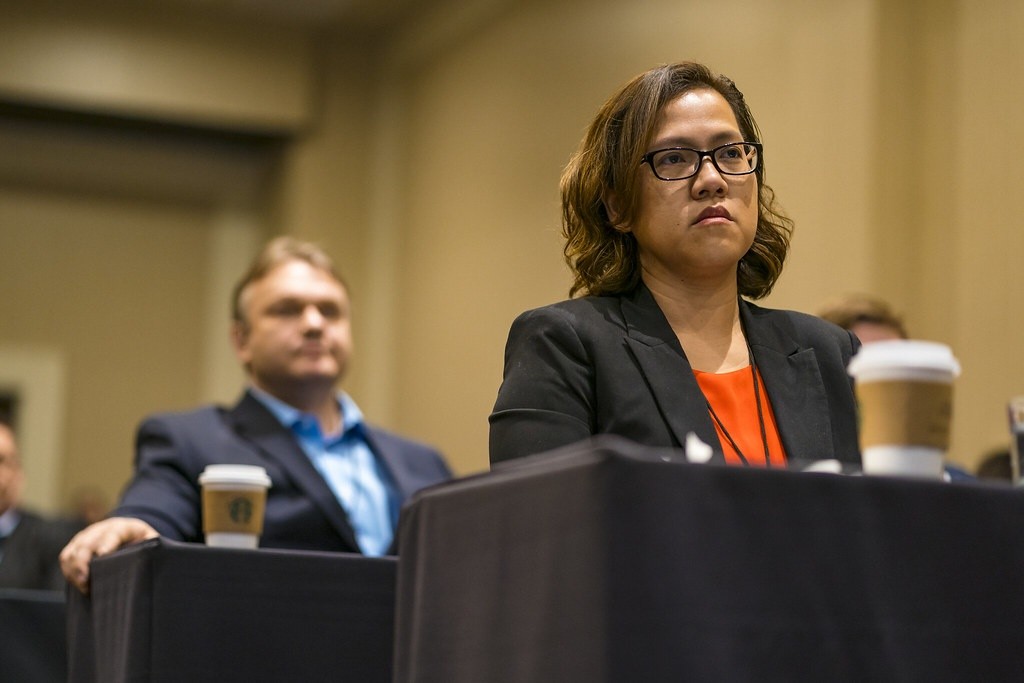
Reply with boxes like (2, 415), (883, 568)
(1007, 399), (1024, 485)
(847, 340), (959, 481)
(198, 465), (272, 549)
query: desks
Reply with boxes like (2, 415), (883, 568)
(0, 437), (1024, 683)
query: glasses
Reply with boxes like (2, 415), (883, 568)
(640, 141), (763, 180)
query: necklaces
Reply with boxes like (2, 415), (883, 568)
(703, 345), (773, 469)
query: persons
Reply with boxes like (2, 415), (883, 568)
(487, 60), (981, 481)
(0, 423), (110, 589)
(57, 234), (457, 594)
